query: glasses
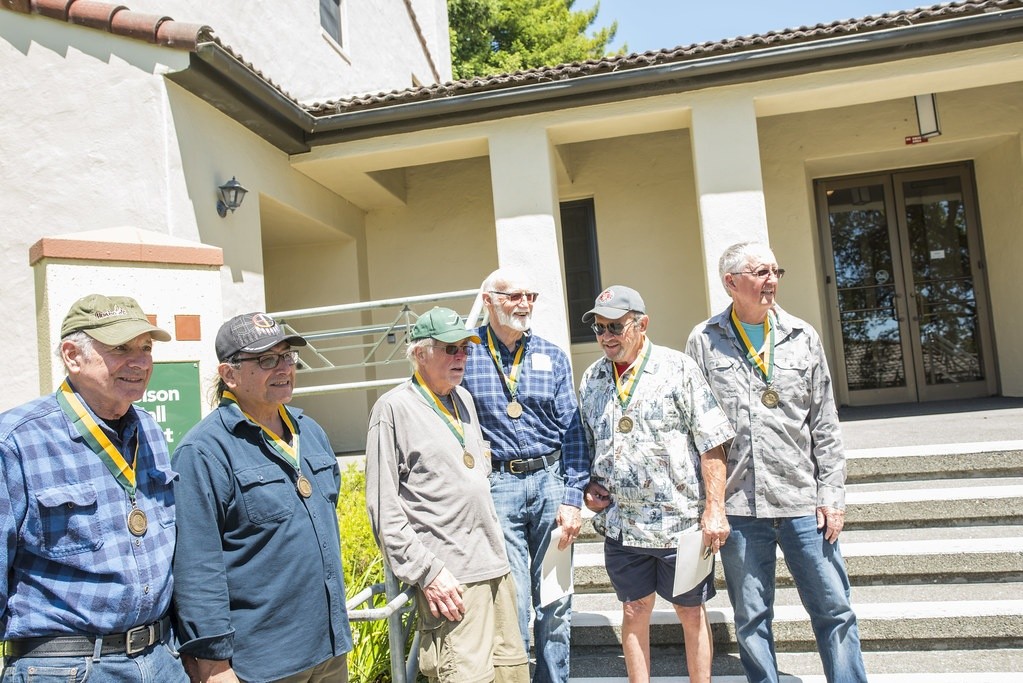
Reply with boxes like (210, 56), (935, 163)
(233, 350), (300, 371)
(429, 344), (474, 356)
(590, 318), (635, 336)
(489, 290), (539, 304)
(730, 269), (785, 280)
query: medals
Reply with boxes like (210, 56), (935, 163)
(169, 312), (353, 683)
(761, 390), (779, 408)
(127, 508), (147, 536)
(506, 402), (522, 419)
(297, 476), (312, 498)
(463, 453), (474, 469)
(618, 416), (633, 434)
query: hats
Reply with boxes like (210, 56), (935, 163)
(61, 294), (171, 347)
(410, 306), (481, 345)
(215, 311), (307, 362)
(581, 285), (646, 324)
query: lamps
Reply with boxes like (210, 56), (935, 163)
(217, 176), (248, 217)
(914, 93), (941, 139)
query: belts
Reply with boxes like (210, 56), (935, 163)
(491, 450), (561, 473)
(6, 613), (172, 657)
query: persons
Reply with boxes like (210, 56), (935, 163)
(365, 305), (530, 683)
(685, 240), (868, 683)
(579, 283), (738, 683)
(461, 267), (591, 683)
(0, 294), (191, 683)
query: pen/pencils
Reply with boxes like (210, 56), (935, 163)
(703, 548), (711, 560)
